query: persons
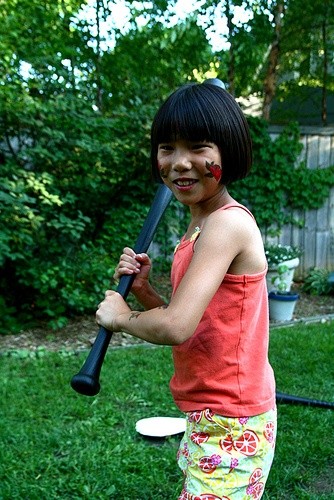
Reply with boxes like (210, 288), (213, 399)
(95, 83), (277, 500)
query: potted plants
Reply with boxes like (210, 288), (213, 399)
(267, 266), (300, 322)
(227, 114), (334, 293)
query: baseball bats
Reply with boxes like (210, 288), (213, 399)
(71, 78), (226, 397)
